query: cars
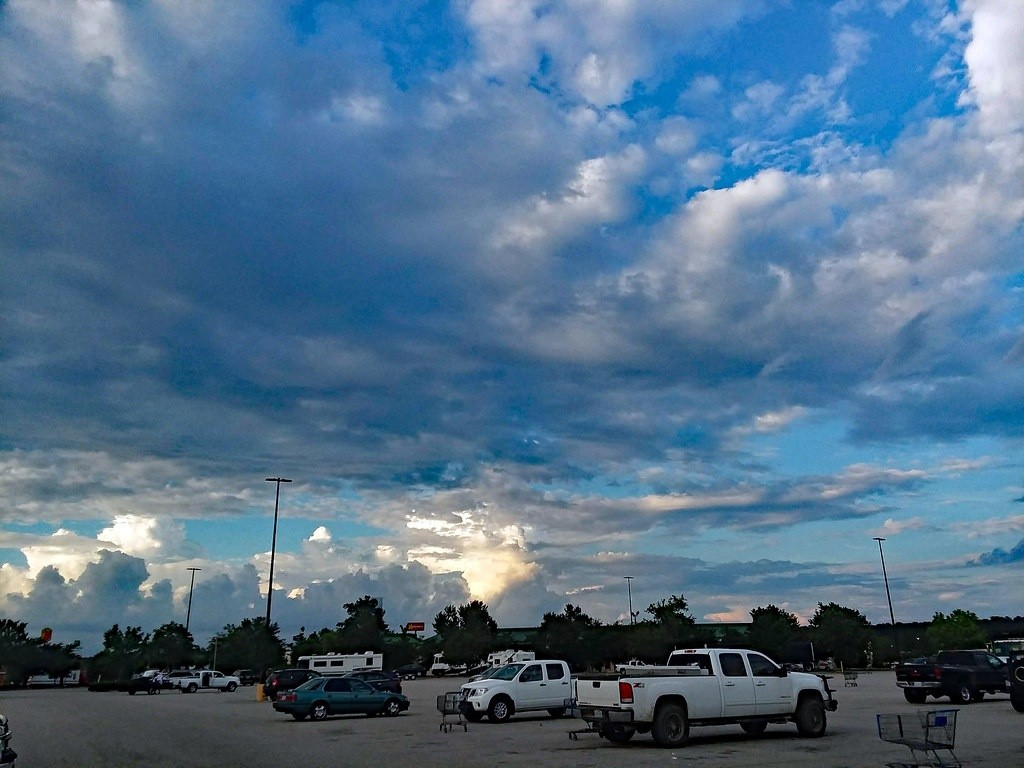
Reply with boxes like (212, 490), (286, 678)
(468, 664), (502, 684)
(0, 715), (18, 768)
(273, 676), (410, 721)
(159, 669), (196, 688)
(229, 669), (257, 686)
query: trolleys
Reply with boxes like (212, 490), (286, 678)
(875, 709), (963, 768)
(842, 668), (858, 687)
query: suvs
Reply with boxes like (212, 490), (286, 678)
(393, 664), (428, 681)
(341, 671), (403, 695)
(262, 668), (323, 701)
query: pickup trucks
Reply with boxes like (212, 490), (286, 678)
(179, 670), (240, 694)
(458, 659), (578, 724)
(895, 649), (1011, 705)
(574, 647), (838, 752)
(614, 659), (649, 675)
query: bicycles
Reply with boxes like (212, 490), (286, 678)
(148, 679), (161, 695)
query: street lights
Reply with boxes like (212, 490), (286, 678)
(622, 575), (640, 625)
(186, 568), (202, 630)
(260, 477), (293, 685)
(872, 537), (895, 626)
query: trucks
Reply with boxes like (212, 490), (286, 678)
(296, 652), (383, 673)
